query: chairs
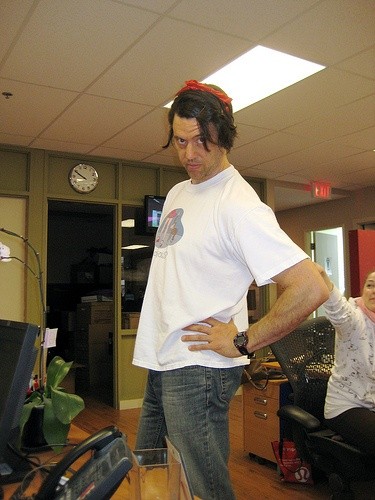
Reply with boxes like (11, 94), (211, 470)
(266, 319), (375, 500)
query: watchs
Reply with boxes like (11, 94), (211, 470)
(234, 331), (255, 359)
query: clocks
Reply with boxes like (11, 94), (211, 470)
(69, 163), (100, 194)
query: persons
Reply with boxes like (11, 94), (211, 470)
(132, 80), (331, 500)
(315, 261), (375, 456)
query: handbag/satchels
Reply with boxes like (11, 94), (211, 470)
(241, 360), (287, 390)
(271, 434), (315, 485)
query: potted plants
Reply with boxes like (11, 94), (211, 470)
(20, 356), (88, 454)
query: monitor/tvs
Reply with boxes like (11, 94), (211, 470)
(144, 194), (166, 234)
(0, 319), (38, 485)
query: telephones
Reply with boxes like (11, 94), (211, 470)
(36, 425), (133, 500)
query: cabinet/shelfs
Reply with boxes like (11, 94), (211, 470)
(241, 382), (282, 476)
